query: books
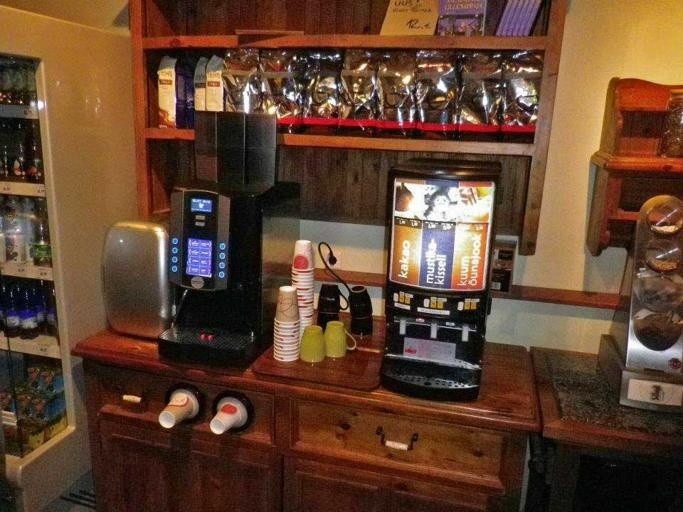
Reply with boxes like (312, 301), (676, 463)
(378, 0), (441, 37)
(437, 1), (488, 36)
(494, 1), (542, 37)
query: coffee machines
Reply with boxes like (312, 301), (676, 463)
(374, 158), (506, 406)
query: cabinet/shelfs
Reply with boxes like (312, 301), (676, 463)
(586, 77), (683, 256)
(529, 346), (682, 510)
(125, 0), (569, 256)
(0, 5), (143, 509)
(70, 311), (543, 511)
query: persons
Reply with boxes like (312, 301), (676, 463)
(423, 190), (457, 223)
(394, 184), (419, 221)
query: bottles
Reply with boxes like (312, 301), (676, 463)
(655, 87), (683, 159)
(0, 52), (58, 343)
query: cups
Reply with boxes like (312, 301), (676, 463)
(208, 397), (249, 436)
(270, 282), (302, 364)
(346, 283), (376, 336)
(158, 389), (200, 433)
(317, 281), (351, 333)
(325, 320), (358, 359)
(290, 238), (316, 353)
(299, 323), (326, 364)
